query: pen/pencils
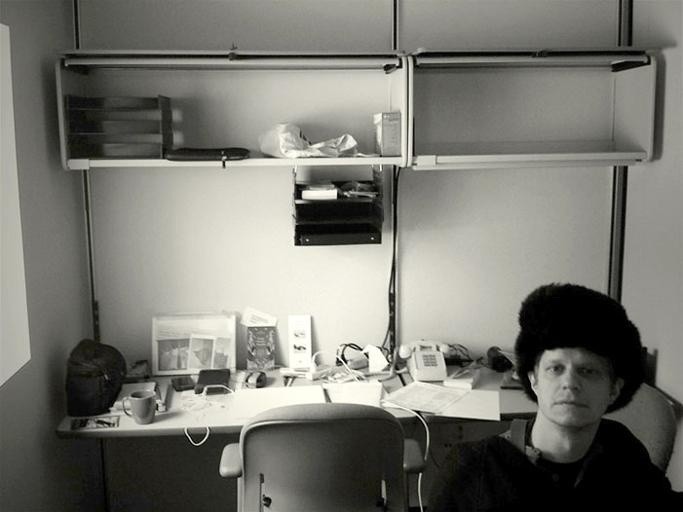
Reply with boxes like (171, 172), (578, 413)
(164, 385), (172, 405)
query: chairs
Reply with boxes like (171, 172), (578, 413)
(602, 383), (677, 472)
(220, 403), (425, 512)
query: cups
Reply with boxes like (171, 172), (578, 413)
(122, 390), (155, 424)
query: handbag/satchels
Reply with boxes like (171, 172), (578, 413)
(66, 339), (126, 417)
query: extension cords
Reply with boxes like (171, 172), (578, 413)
(306, 356), (367, 380)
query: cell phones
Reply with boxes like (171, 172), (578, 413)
(172, 376), (194, 391)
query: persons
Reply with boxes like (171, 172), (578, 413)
(415, 283), (682, 512)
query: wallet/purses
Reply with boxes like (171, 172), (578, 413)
(167, 148), (250, 168)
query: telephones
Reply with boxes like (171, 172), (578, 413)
(398, 340), (449, 382)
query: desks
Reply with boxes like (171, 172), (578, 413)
(55, 359), (537, 511)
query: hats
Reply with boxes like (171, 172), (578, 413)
(516, 284), (645, 414)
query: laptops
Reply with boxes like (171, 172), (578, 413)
(247, 371), (267, 387)
